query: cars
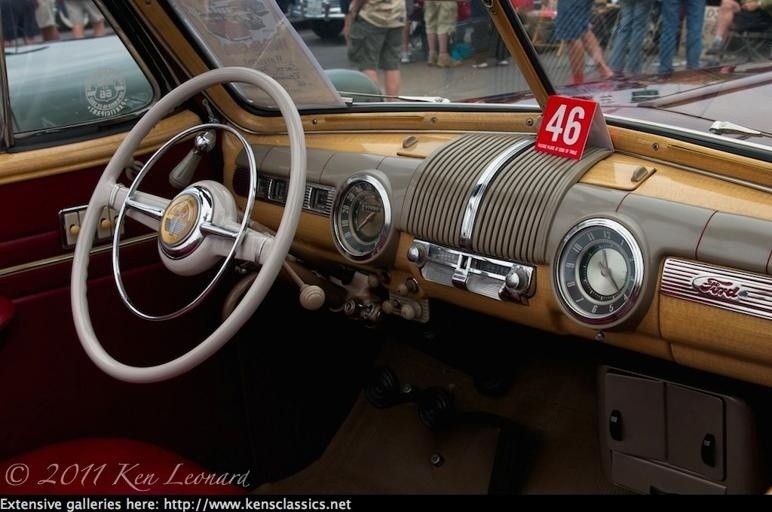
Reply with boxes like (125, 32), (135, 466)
(3, 33), (392, 135)
(430, 1), (560, 66)
(15, 1), (109, 34)
(288, 0), (353, 39)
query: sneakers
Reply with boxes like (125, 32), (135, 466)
(426, 54), (461, 67)
(400, 51), (411, 62)
(471, 61), (509, 68)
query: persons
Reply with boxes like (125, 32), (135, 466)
(1, 0), (108, 47)
(339, 0), (771, 102)
